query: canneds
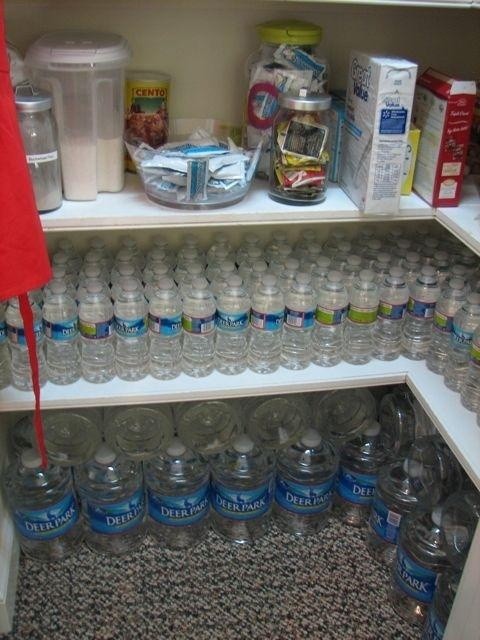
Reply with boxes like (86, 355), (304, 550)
(125, 70), (171, 173)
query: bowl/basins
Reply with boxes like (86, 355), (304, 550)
(123, 117), (265, 212)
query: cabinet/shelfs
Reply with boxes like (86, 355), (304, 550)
(0, 5), (480, 640)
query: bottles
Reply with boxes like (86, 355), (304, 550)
(244, 13), (330, 178)
(11, 34), (65, 216)
(25, 27), (131, 201)
(268, 89), (330, 205)
(2, 384), (480, 640)
(0, 226), (480, 425)
